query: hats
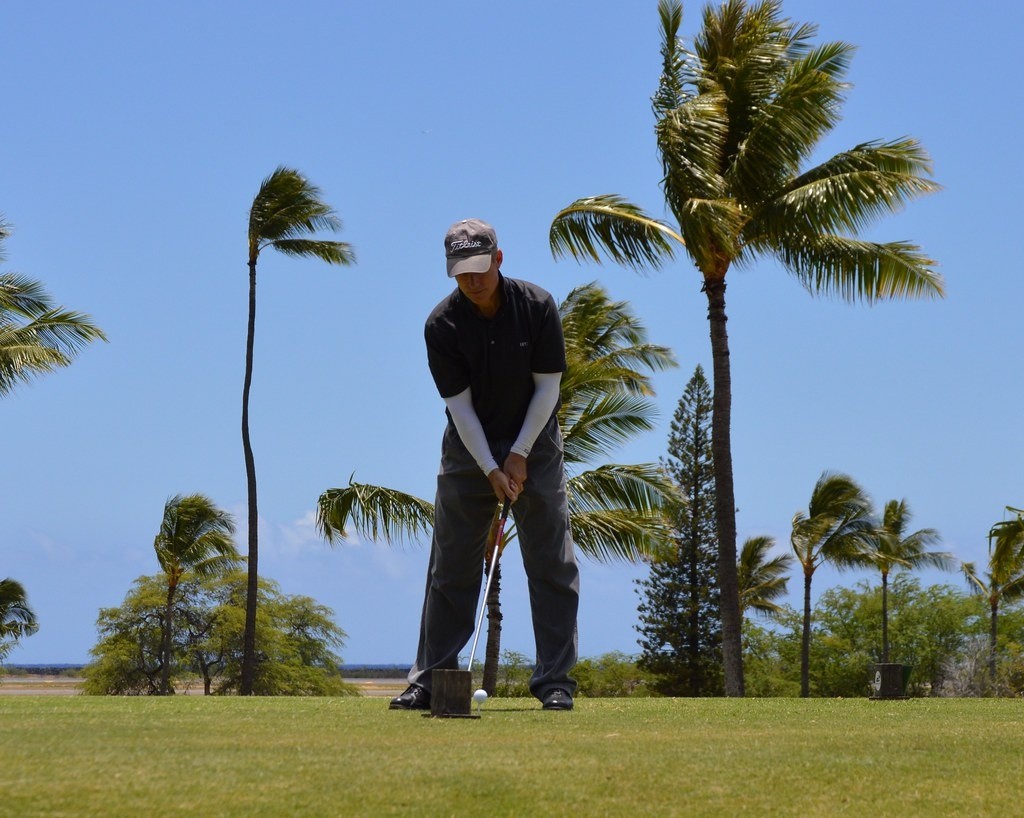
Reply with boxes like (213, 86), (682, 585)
(444, 219), (497, 277)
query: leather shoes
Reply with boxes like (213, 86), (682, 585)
(389, 683), (431, 711)
(542, 689), (573, 711)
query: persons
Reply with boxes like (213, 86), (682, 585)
(389, 216), (580, 710)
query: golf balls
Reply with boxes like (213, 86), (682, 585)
(473, 689), (488, 704)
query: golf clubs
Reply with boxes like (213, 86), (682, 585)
(467, 492), (511, 673)
